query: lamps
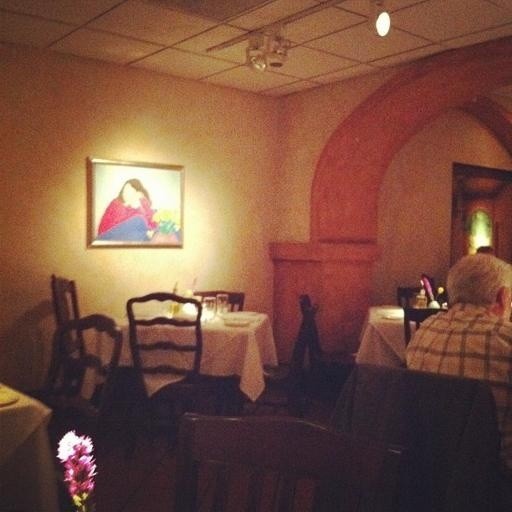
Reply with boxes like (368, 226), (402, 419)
(206, 0), (391, 71)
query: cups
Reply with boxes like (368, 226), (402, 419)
(202, 294), (229, 321)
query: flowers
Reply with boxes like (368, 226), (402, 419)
(57, 430), (99, 512)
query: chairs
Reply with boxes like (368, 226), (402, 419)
(229, 302), (320, 416)
(126, 292), (225, 414)
(396, 285), (447, 306)
(402, 302), (448, 347)
(297, 294), (352, 402)
(166, 410), (411, 512)
(330, 361), (512, 512)
(194, 291), (245, 310)
(28, 312), (123, 463)
(50, 273), (113, 401)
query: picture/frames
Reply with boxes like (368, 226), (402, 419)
(86, 155), (186, 249)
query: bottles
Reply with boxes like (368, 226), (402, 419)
(168, 289), (181, 320)
(416, 290), (427, 310)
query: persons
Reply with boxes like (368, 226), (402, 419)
(404, 252), (512, 485)
(96, 177), (160, 242)
